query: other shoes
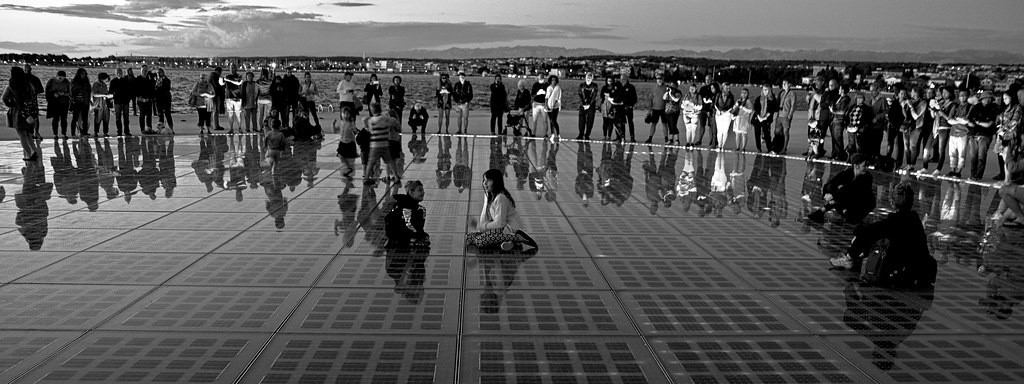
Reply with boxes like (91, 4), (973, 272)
(409, 240), (432, 249)
(500, 241), (515, 251)
(806, 209), (826, 224)
(12, 126), (1022, 190)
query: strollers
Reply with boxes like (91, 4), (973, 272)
(503, 106), (532, 136)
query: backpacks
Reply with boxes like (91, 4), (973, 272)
(864, 239), (892, 280)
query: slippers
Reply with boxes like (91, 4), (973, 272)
(1002, 217), (1024, 226)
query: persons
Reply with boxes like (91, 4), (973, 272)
(472, 248), (538, 315)
(356, 181), (374, 241)
(916, 179), (1024, 323)
(828, 180), (929, 285)
(361, 181), (387, 257)
(465, 168), (538, 251)
(7, 133), (321, 252)
(829, 266), (927, 371)
(407, 132), (828, 234)
(803, 216), (873, 257)
(384, 180), (430, 249)
(384, 246), (432, 305)
(998, 130), (1024, 228)
(806, 152), (878, 227)
(4, 64), (1022, 188)
(383, 179), (399, 225)
(333, 183), (360, 247)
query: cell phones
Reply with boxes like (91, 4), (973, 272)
(666, 85), (669, 92)
(373, 81), (379, 85)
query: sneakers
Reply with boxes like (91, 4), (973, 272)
(829, 254), (854, 269)
